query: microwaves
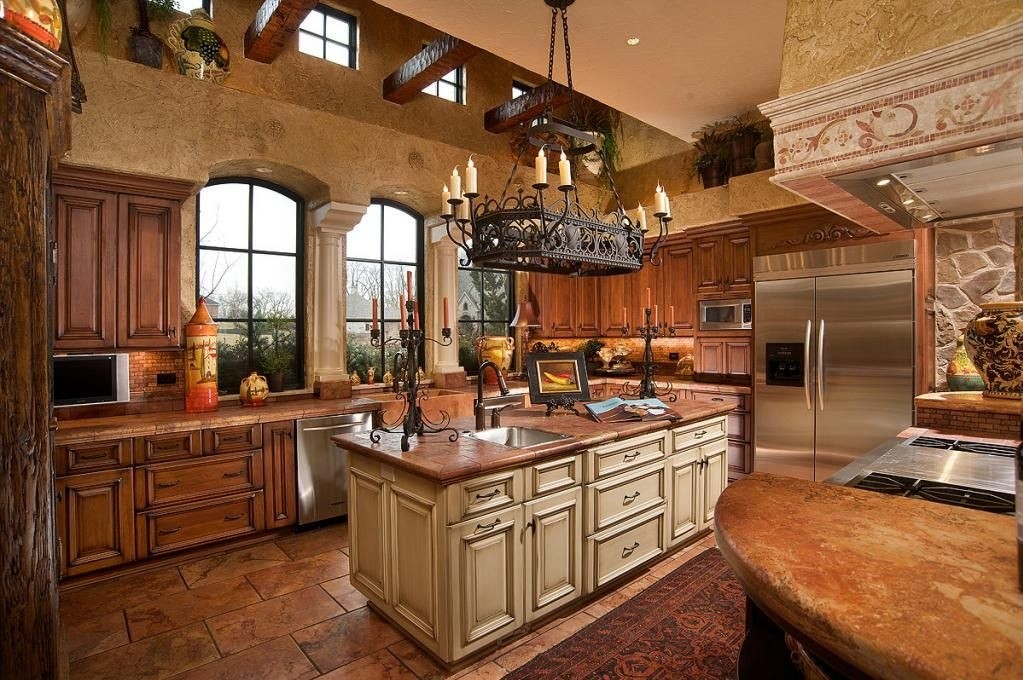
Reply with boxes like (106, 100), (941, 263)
(52, 351), (131, 410)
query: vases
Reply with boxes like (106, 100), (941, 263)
(473, 336), (515, 385)
(964, 301), (1022, 399)
(946, 335), (984, 391)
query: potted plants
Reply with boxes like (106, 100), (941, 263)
(95, 1), (175, 70)
(755, 119), (774, 170)
(688, 130), (727, 190)
(691, 113), (759, 176)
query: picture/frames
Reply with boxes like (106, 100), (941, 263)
(525, 351), (592, 404)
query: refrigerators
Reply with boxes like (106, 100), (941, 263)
(749, 233), (918, 484)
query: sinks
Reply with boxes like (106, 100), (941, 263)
(463, 424), (576, 449)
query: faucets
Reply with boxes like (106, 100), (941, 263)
(475, 361), (509, 430)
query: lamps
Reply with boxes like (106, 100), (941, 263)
(440, 1), (672, 278)
(509, 300), (542, 381)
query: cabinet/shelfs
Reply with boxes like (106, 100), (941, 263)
(56, 384), (480, 582)
(714, 469), (1023, 678)
(53, 162), (191, 354)
(580, 285), (755, 481)
(329, 394), (737, 675)
(529, 219), (754, 337)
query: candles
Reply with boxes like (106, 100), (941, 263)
(653, 304), (657, 327)
(407, 271), (412, 301)
(444, 296), (449, 328)
(400, 295), (407, 330)
(373, 298), (378, 330)
(414, 302), (420, 330)
(623, 308), (626, 327)
(669, 306), (674, 327)
(646, 287), (650, 309)
(639, 307), (644, 329)
